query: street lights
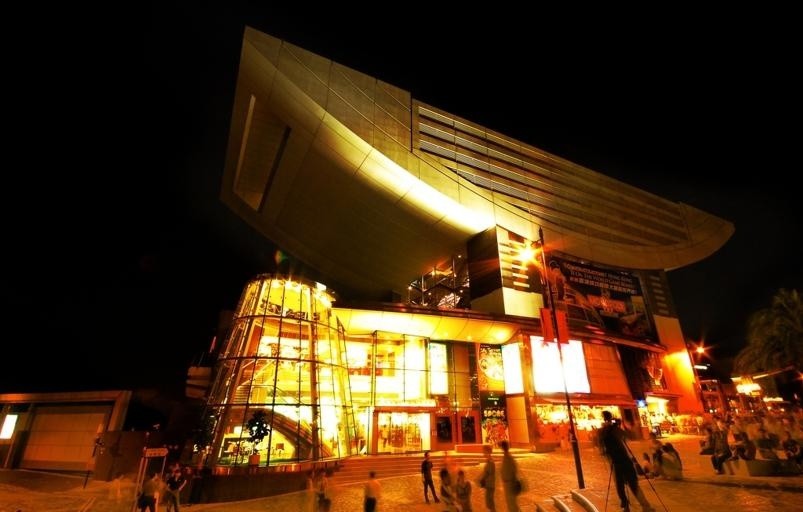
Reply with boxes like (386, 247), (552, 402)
(518, 226), (584, 491)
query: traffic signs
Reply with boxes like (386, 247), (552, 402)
(146, 448), (169, 457)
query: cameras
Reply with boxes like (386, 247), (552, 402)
(611, 418), (621, 426)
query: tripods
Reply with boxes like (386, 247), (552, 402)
(605, 438), (668, 512)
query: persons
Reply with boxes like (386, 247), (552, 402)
(305, 469), (317, 511)
(479, 445), (496, 512)
(316, 469), (328, 502)
(597, 411), (655, 511)
(363, 471), (381, 512)
(455, 471), (473, 512)
(500, 439), (520, 512)
(320, 498), (331, 511)
(637, 409), (803, 481)
(108, 463), (205, 511)
(589, 422), (606, 457)
(440, 468), (459, 512)
(420, 451), (441, 503)
